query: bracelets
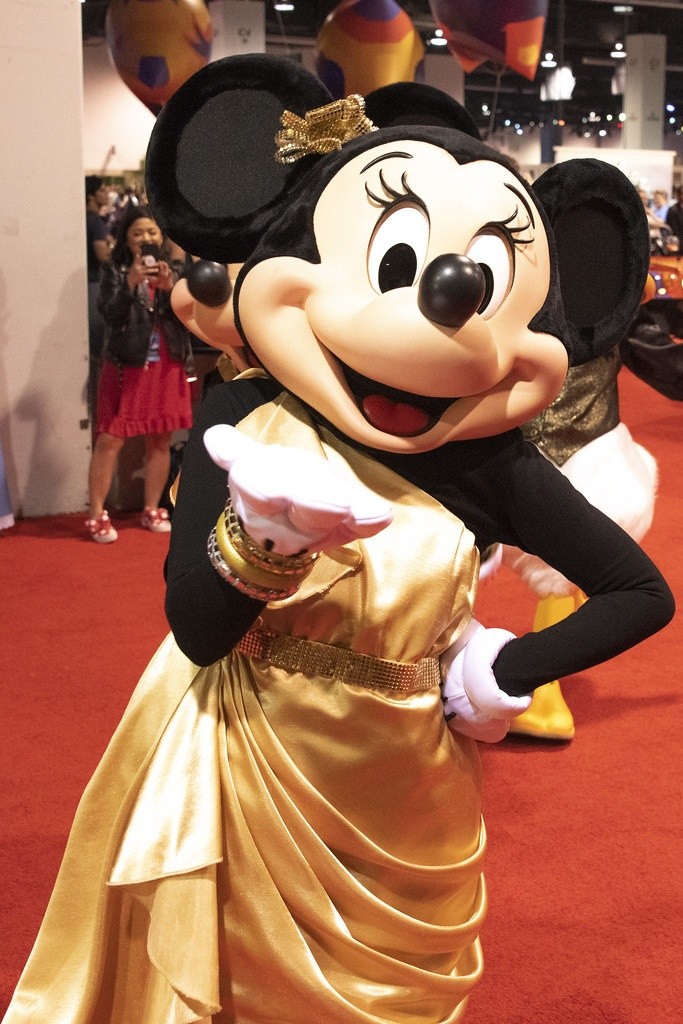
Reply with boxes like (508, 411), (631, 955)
(207, 497), (322, 602)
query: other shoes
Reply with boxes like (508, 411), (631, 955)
(84, 509), (118, 544)
(141, 507), (171, 532)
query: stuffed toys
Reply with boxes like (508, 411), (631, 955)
(2, 52), (677, 1024)
(169, 79), (665, 740)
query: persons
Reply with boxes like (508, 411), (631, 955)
(84, 175), (203, 545)
(634, 181), (683, 256)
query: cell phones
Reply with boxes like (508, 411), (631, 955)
(140, 245), (160, 277)
